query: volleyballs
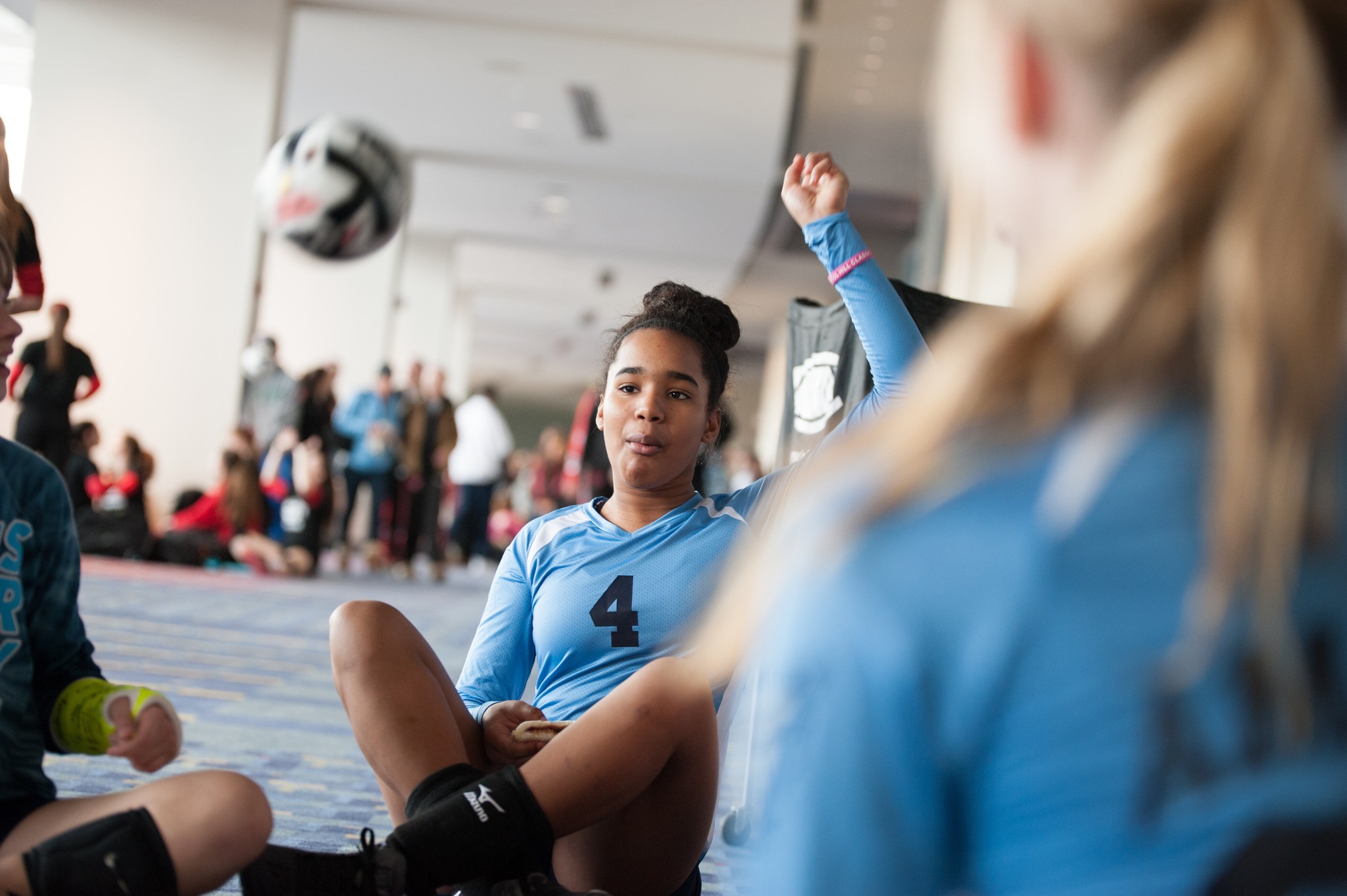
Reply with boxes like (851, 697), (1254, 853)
(258, 116), (413, 260)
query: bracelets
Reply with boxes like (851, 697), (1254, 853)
(827, 248), (872, 286)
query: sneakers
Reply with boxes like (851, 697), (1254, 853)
(405, 763), (608, 896)
(238, 765), (557, 896)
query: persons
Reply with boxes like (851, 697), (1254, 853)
(688, 1), (1347, 896)
(1, 119), (936, 896)
